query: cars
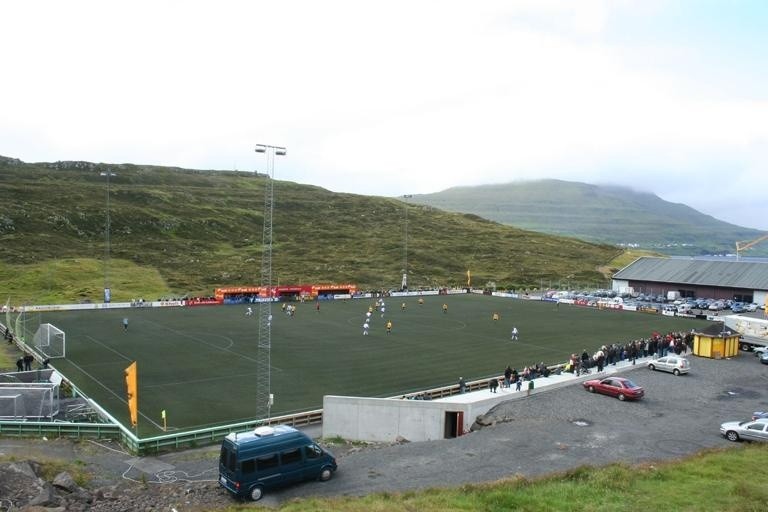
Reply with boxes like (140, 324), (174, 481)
(582, 376), (645, 401)
(753, 345), (768, 364)
(720, 411), (768, 445)
(647, 355), (691, 376)
(543, 287), (767, 314)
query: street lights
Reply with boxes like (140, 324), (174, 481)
(101, 172), (116, 288)
(404, 194), (412, 273)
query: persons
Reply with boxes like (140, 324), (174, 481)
(418, 298), (425, 305)
(458, 377), (466, 393)
(493, 312), (499, 321)
(403, 392), (432, 400)
(568, 330), (689, 377)
(511, 327), (519, 341)
(247, 305), (252, 316)
(16, 356), (24, 372)
(443, 304), (448, 313)
(23, 351), (34, 371)
(316, 301), (320, 312)
(400, 303), (408, 313)
(362, 295), (395, 337)
(123, 317), (129, 332)
(267, 313), (273, 327)
(489, 361), (551, 395)
(281, 303), (296, 317)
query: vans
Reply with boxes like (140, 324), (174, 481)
(217, 424), (338, 502)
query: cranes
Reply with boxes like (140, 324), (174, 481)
(736, 233), (768, 260)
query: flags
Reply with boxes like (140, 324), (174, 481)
(123, 362), (138, 427)
